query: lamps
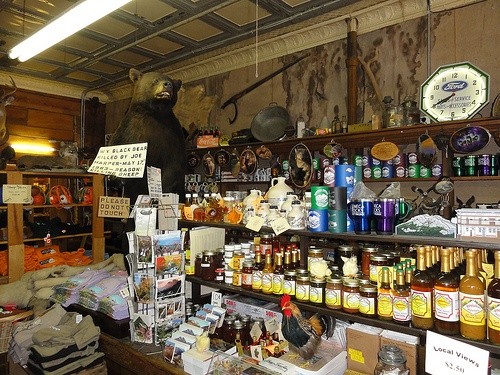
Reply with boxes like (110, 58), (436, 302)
(6, 0), (131, 63)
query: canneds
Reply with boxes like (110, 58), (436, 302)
(453, 154), (499, 176)
(284, 246), (401, 318)
(224, 313), (267, 346)
(282, 153), (442, 178)
(195, 233), (288, 289)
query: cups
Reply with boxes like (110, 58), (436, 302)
(452, 154), (500, 176)
(254, 168), (271, 182)
(309, 153), (443, 237)
(184, 182), (218, 193)
(225, 190), (247, 201)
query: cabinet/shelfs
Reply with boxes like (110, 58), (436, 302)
(46, 117), (500, 375)
(0, 170), (111, 285)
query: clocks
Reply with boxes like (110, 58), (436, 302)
(418, 61), (490, 123)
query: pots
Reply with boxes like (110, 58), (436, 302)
(251, 102), (289, 142)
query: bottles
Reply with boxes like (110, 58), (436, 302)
(251, 244), (301, 297)
(198, 126), (220, 138)
(382, 101), (420, 128)
(331, 116), (348, 134)
(459, 248), (494, 341)
(410, 245), (443, 329)
(433, 247), (466, 335)
(296, 113), (331, 138)
(223, 197), (235, 223)
(201, 193), (220, 222)
(181, 193), (200, 221)
(487, 250), (500, 344)
(377, 259), (411, 321)
(392, 265), (417, 325)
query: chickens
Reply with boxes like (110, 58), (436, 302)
(310, 261), (332, 278)
(280, 294), (336, 367)
(342, 256), (359, 277)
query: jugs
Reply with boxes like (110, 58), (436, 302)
(242, 177), (311, 230)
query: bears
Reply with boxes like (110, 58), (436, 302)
(102, 69), (200, 231)
